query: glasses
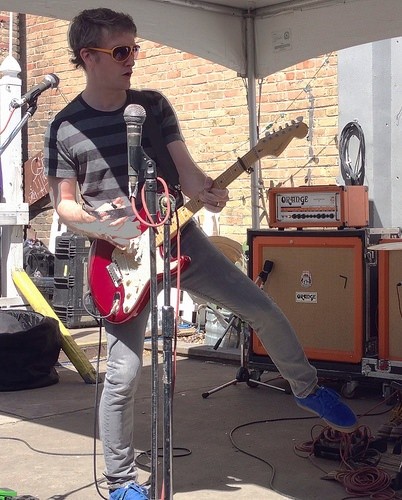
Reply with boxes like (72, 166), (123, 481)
(84, 46), (140, 63)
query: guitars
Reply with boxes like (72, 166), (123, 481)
(87, 115), (308, 325)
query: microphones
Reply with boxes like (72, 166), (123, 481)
(123, 104), (147, 199)
(13, 73), (59, 109)
(258, 260), (273, 290)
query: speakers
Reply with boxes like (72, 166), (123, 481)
(253, 236), (364, 364)
(378, 239), (402, 362)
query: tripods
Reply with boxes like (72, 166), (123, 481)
(201, 271), (291, 398)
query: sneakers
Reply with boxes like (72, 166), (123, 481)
(294, 385), (358, 434)
(110, 483), (148, 500)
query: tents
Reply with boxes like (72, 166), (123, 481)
(0, 0), (402, 252)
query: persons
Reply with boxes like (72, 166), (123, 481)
(45, 8), (358, 500)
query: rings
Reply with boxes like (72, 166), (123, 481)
(216, 202), (219, 207)
